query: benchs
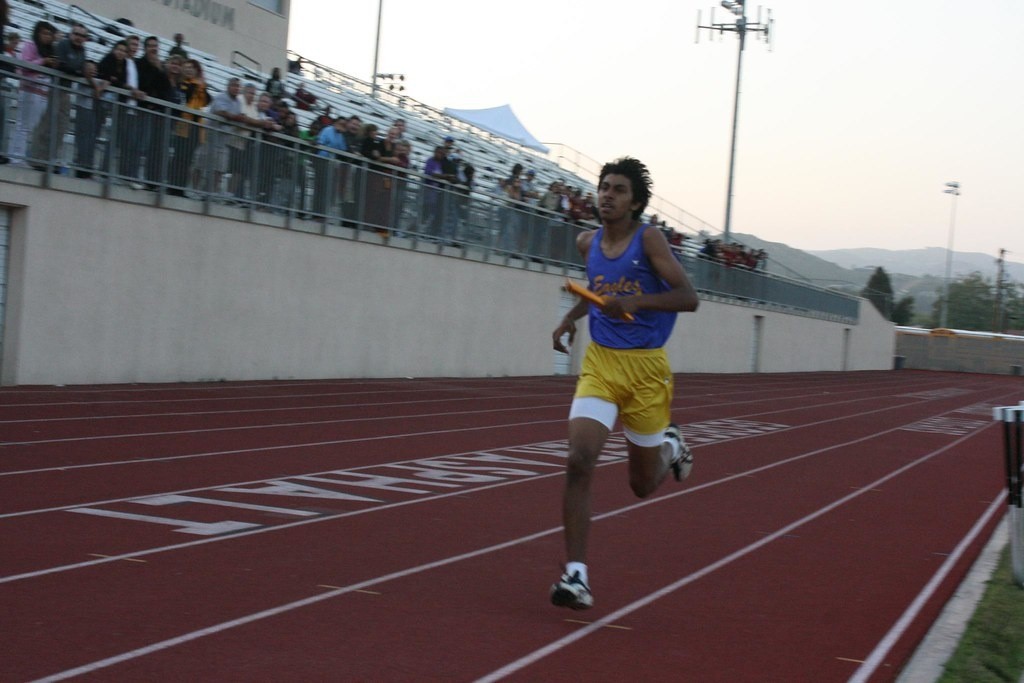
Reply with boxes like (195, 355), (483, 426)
(1, 1), (707, 286)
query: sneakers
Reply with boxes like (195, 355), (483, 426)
(550, 563), (594, 610)
(664, 422), (694, 482)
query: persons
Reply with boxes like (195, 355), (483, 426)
(0, 0), (769, 272)
(549, 157), (700, 610)
